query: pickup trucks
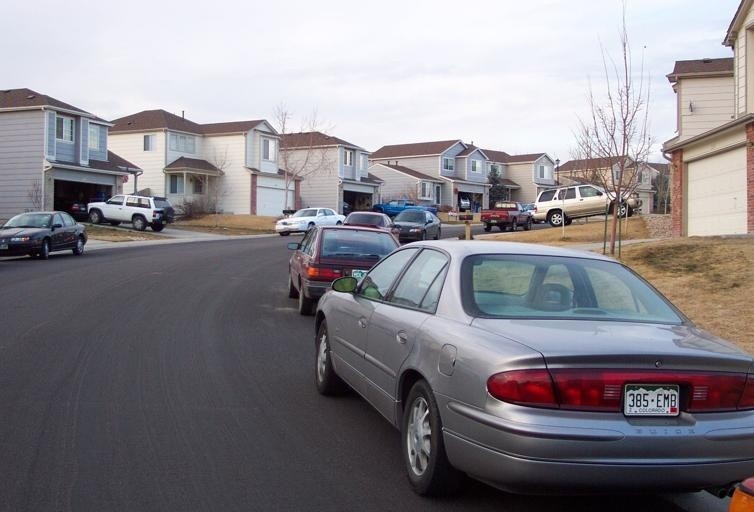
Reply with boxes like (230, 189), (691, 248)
(371, 198), (439, 217)
(479, 200), (533, 232)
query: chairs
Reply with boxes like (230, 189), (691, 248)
(535, 281), (571, 311)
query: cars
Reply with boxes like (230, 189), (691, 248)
(54, 197), (89, 222)
(524, 184), (643, 228)
(312, 236), (754, 502)
(274, 206), (442, 316)
(0, 209), (89, 260)
(459, 195), (471, 211)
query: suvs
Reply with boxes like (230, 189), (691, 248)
(84, 192), (176, 232)
